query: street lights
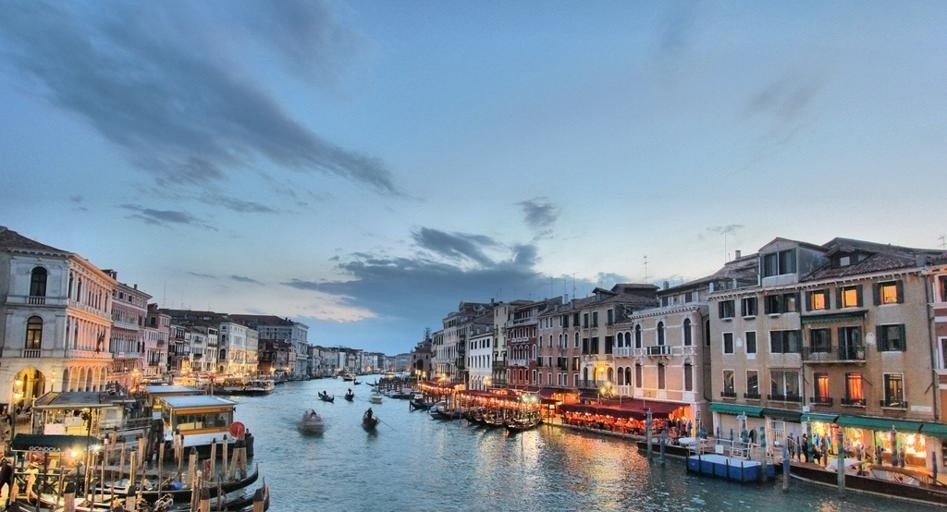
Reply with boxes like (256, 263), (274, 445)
(132, 367), (138, 386)
(600, 382), (615, 402)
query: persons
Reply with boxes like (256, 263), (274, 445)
(0, 457), (87, 498)
(367, 407), (373, 420)
(661, 417), (809, 465)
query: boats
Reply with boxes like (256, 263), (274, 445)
(316, 369), (545, 439)
(300, 411), (325, 435)
(0, 373), (277, 512)
